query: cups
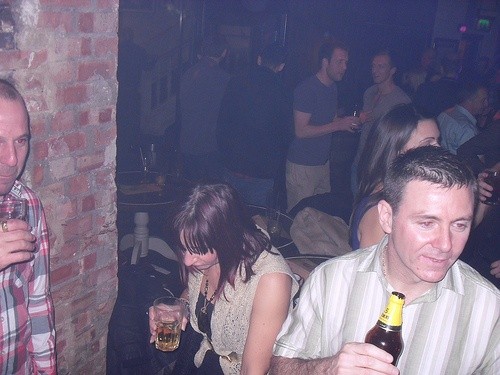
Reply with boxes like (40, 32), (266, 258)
(266, 212), (279, 233)
(153, 297), (183, 351)
(481, 169), (500, 207)
(0, 198), (28, 221)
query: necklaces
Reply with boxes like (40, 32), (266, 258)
(201, 271), (224, 315)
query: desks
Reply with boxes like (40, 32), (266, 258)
(242, 205), (342, 308)
(114, 170), (197, 264)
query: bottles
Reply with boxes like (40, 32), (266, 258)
(351, 104), (360, 134)
(365, 291), (406, 366)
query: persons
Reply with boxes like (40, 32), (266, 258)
(272, 146), (500, 374)
(174, 31), (499, 288)
(147, 181), (301, 375)
(0, 80), (58, 375)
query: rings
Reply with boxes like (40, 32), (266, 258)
(2, 222), (7, 230)
